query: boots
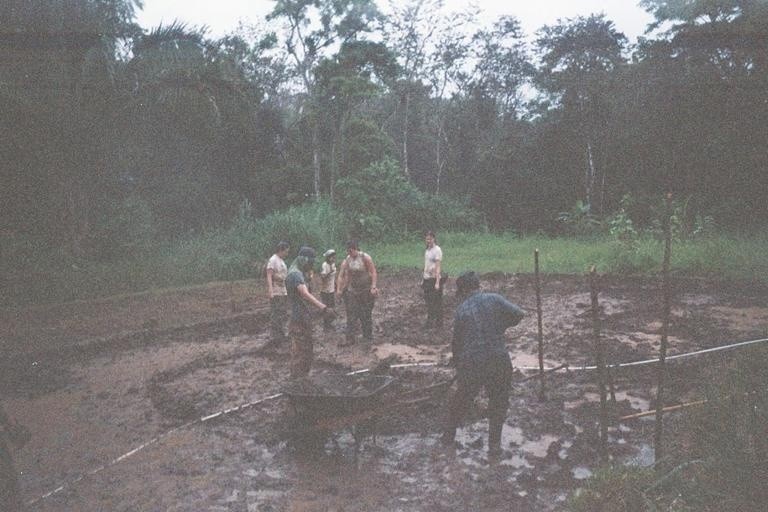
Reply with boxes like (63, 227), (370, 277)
(437, 412), (458, 444)
(338, 329), (373, 350)
(486, 413), (505, 457)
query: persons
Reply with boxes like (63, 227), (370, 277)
(320, 249), (337, 330)
(439, 271), (524, 456)
(285, 246), (336, 377)
(267, 242), (290, 347)
(418, 231), (443, 327)
(336, 240), (378, 346)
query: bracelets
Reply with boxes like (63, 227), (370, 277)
(321, 307), (327, 314)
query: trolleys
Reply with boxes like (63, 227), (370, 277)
(275, 369), (447, 479)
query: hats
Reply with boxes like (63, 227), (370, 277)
(323, 248), (336, 257)
(346, 239), (359, 252)
(454, 270), (480, 297)
(299, 247), (316, 258)
